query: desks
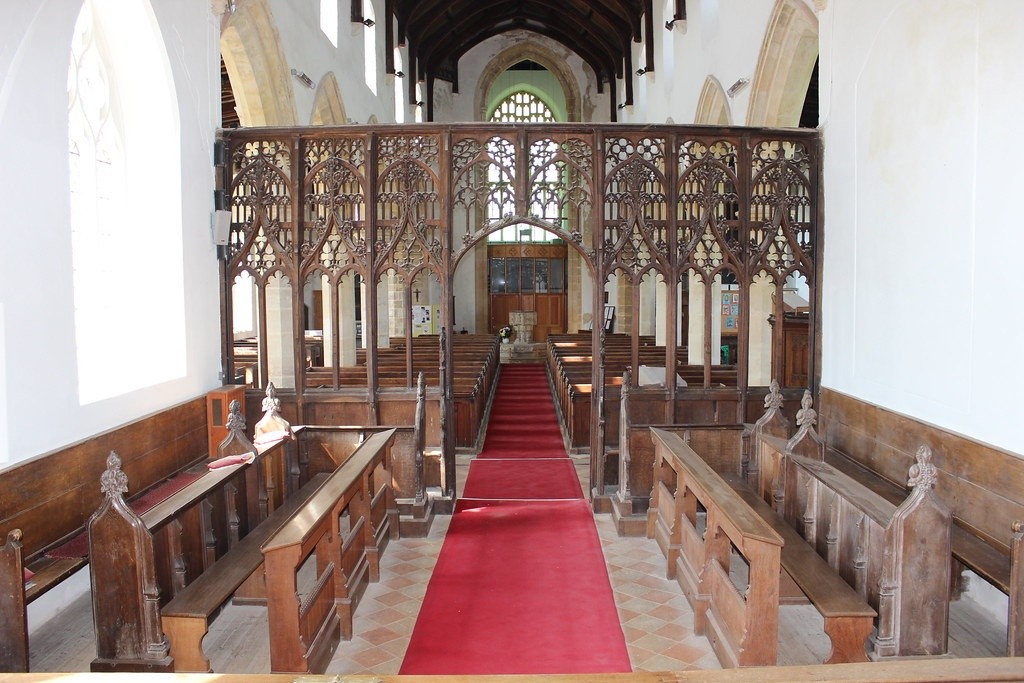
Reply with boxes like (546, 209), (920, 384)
(766, 311), (810, 388)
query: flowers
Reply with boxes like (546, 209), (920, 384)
(499, 326), (514, 339)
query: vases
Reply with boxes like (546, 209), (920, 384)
(502, 338), (509, 343)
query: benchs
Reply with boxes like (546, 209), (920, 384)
(234, 330), (323, 388)
(0, 387), (207, 673)
(546, 333), (738, 449)
(306, 334), (500, 448)
(817, 385), (1024, 657)
(160, 472), (356, 673)
(716, 472), (879, 664)
